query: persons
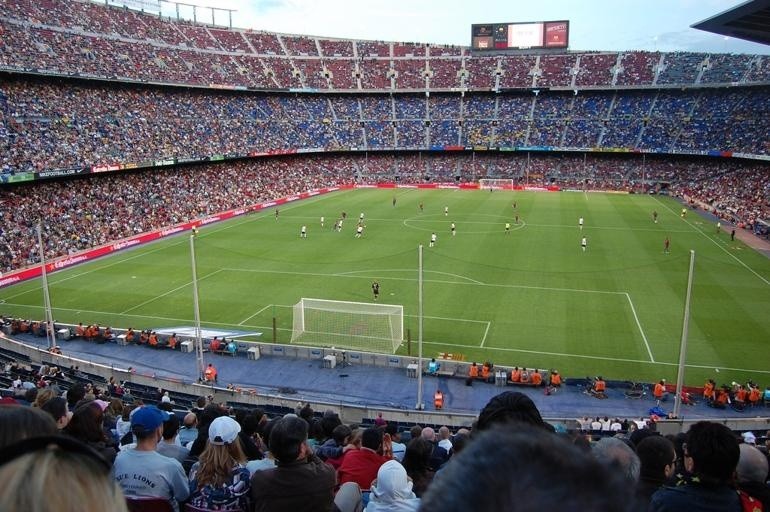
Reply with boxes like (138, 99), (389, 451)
(1, 1), (770, 288)
(371, 279), (380, 302)
(0, 314), (770, 511)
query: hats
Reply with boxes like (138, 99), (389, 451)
(208, 416), (242, 446)
(129, 404), (170, 433)
(741, 432), (756, 444)
